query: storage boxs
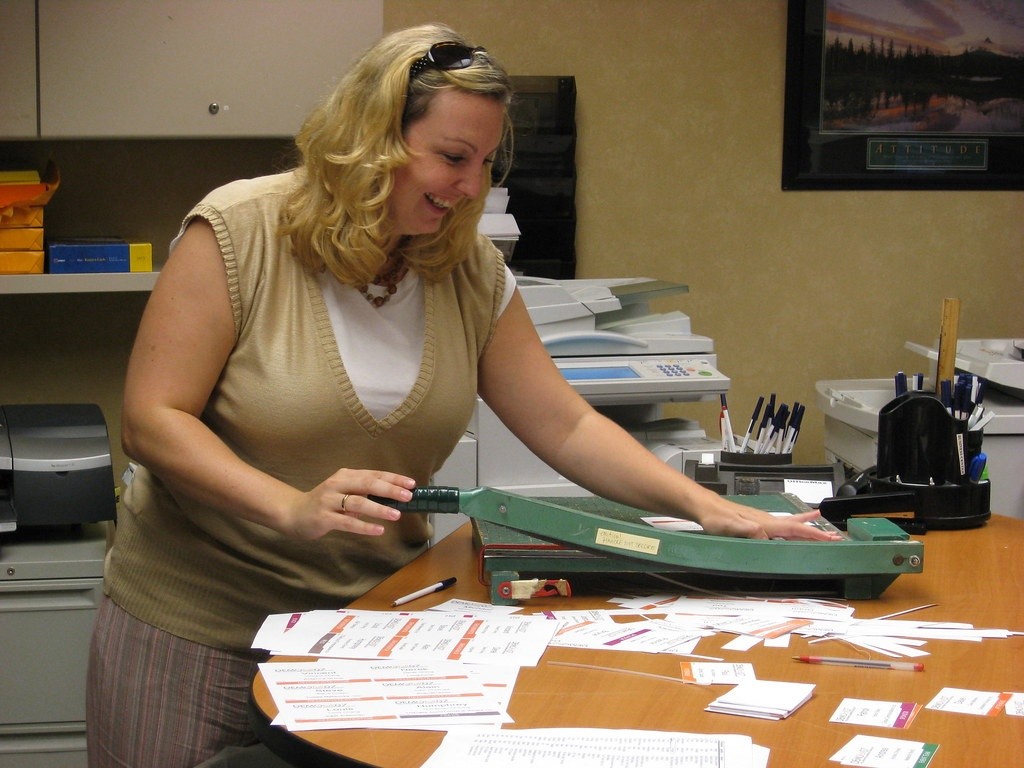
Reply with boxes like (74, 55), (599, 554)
(44, 236), (152, 273)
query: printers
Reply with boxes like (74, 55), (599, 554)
(0, 402), (115, 532)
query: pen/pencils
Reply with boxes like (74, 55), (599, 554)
(892, 369), (996, 486)
(789, 658), (926, 672)
(390, 576), (458, 604)
(716, 393), (806, 464)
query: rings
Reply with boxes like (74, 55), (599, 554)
(342, 494), (349, 512)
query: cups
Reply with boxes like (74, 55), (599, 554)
(720, 451), (793, 465)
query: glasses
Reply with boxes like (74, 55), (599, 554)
(409, 40), (490, 82)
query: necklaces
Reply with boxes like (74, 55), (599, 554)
(357, 257), (404, 306)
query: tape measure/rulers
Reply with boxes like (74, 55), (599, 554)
(934, 297), (958, 396)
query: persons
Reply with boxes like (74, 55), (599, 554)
(85, 22), (846, 768)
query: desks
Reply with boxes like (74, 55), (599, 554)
(252, 514), (1024, 768)
